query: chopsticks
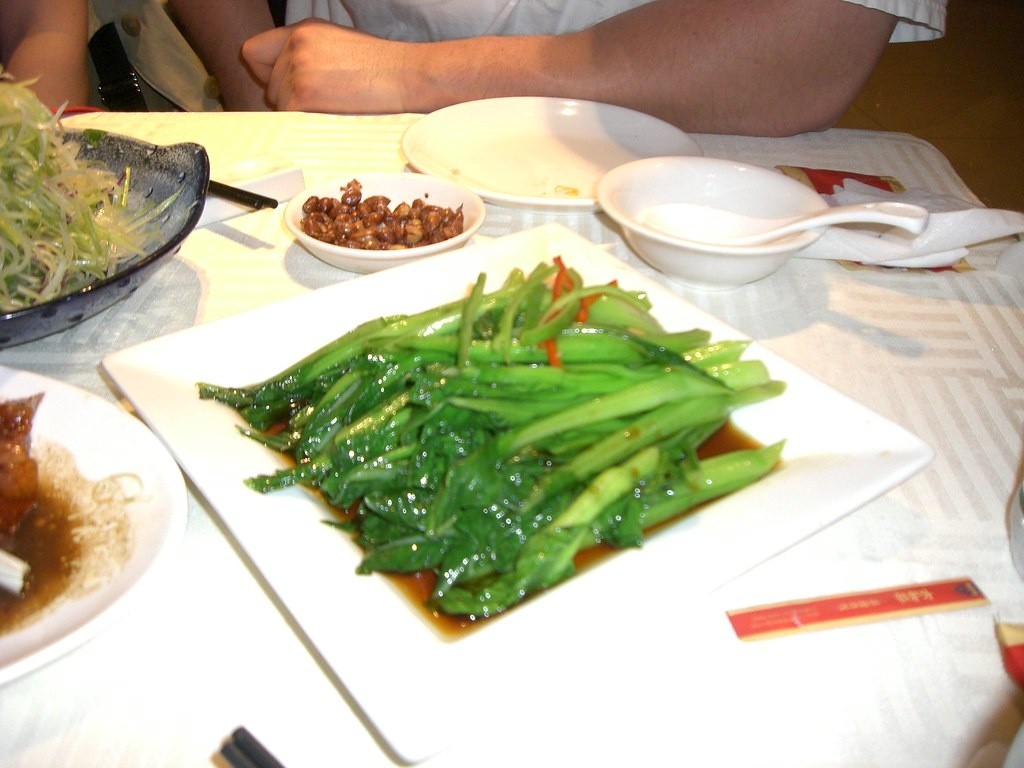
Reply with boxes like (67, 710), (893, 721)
(220, 726), (286, 768)
(207, 179), (278, 210)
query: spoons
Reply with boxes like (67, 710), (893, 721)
(640, 201), (930, 245)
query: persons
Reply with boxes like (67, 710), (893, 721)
(0, 0), (103, 120)
(92, 0), (947, 138)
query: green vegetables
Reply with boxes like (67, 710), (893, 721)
(193, 258), (787, 615)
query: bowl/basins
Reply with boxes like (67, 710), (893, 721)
(284, 172), (485, 275)
(596, 156), (830, 292)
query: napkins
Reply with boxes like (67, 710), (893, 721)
(792, 179), (1023, 269)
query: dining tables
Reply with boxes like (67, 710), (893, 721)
(0, 111), (1023, 768)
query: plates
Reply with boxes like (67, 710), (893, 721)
(0, 128), (210, 349)
(1008, 482), (1024, 582)
(401, 96), (704, 214)
(99, 221), (940, 764)
(0, 364), (187, 686)
(1002, 722), (1024, 768)
